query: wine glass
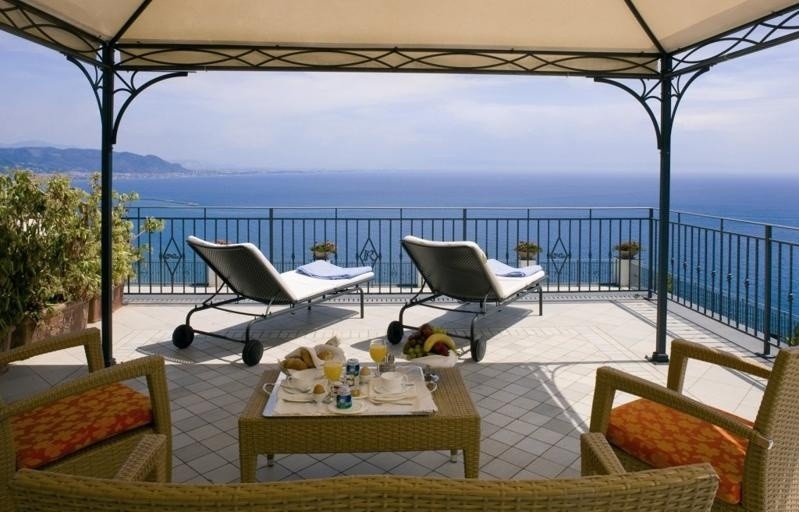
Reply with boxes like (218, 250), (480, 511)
(278, 339), (417, 413)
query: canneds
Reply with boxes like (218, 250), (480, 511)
(346, 358), (360, 376)
(336, 386), (352, 409)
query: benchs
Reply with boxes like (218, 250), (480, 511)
(10, 461), (721, 511)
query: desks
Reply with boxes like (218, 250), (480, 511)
(237, 369), (481, 479)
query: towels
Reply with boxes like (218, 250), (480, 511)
(293, 257), (373, 281)
(482, 257), (543, 279)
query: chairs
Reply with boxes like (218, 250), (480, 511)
(172, 235), (376, 367)
(1, 327), (174, 484)
(386, 235), (546, 363)
(589, 338), (799, 512)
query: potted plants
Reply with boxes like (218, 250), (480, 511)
(512, 240), (543, 260)
(0, 166), (165, 349)
(612, 241), (640, 260)
(310, 239), (336, 260)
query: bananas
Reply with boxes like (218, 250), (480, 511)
(424, 333), (461, 355)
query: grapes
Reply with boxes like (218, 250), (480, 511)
(404, 323), (448, 360)
